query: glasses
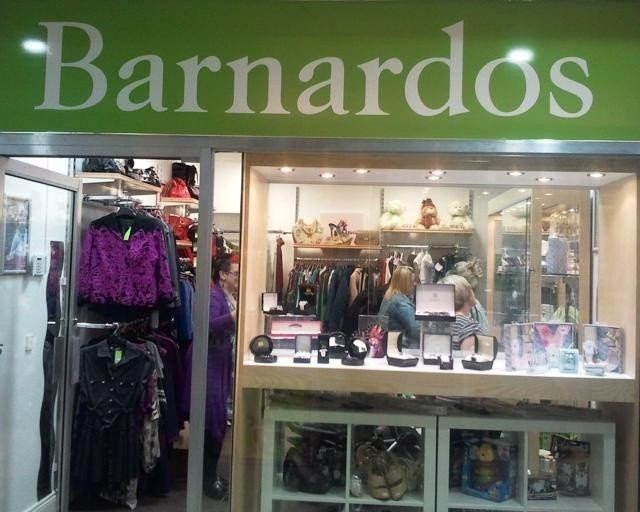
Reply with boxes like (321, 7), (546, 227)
(228, 272), (238, 276)
(400, 266), (414, 272)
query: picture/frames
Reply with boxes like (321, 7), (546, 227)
(2, 195), (31, 275)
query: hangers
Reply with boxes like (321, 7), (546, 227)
(110, 317), (177, 357)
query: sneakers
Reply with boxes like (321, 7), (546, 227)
(206, 480), (225, 500)
(215, 473), (229, 492)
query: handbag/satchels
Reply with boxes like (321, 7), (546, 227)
(292, 218), (324, 244)
(82, 156), (122, 173)
(162, 176), (191, 198)
(188, 185), (199, 200)
(124, 158), (161, 187)
(172, 163), (198, 185)
(169, 214), (193, 240)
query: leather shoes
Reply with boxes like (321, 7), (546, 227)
(283, 424), (423, 494)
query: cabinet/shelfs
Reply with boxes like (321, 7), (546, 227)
(72, 171), (214, 331)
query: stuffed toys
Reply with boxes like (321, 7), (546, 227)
(415, 198), (440, 231)
(380, 199), (403, 230)
(439, 200), (473, 231)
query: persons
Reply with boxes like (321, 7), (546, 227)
(378, 265), (420, 349)
(430, 274), (483, 351)
(445, 257), (488, 337)
(203, 252), (238, 502)
(4, 201), (28, 271)
